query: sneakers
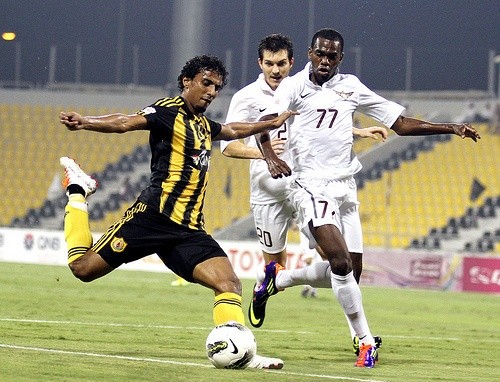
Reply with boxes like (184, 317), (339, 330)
(244, 355), (283, 369)
(254, 261), (287, 305)
(354, 343), (378, 368)
(249, 284), (266, 327)
(353, 335), (382, 356)
(60, 156), (99, 198)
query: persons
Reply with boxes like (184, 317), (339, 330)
(220, 32), (388, 328)
(253, 29), (481, 369)
(60, 55), (300, 371)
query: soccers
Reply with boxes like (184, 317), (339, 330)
(205, 322), (256, 369)
